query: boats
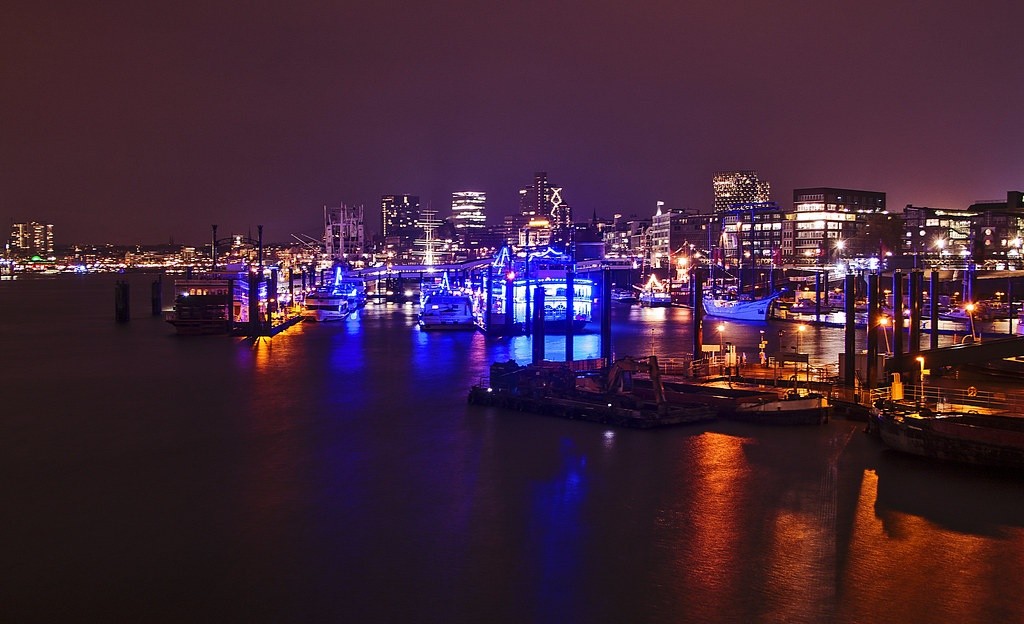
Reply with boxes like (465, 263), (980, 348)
(304, 270), (479, 334)
(610, 271), (673, 308)
(724, 394), (836, 427)
(160, 267), (271, 338)
(784, 292), (1024, 336)
(702, 287), (790, 323)
(869, 404), (1024, 476)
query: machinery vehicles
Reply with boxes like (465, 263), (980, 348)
(573, 354), (671, 418)
